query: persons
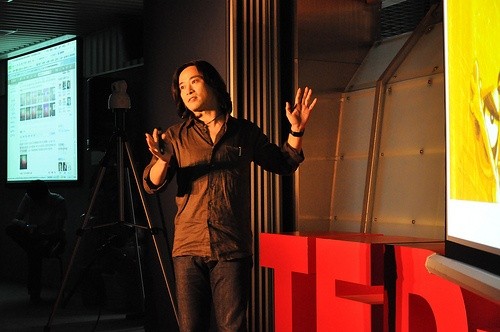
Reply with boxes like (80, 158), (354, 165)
(8, 180), (69, 304)
(142, 61), (317, 332)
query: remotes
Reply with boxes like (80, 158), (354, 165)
(158, 126), (165, 154)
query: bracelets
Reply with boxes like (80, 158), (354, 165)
(290, 128), (305, 137)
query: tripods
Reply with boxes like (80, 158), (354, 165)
(41, 111), (182, 332)
